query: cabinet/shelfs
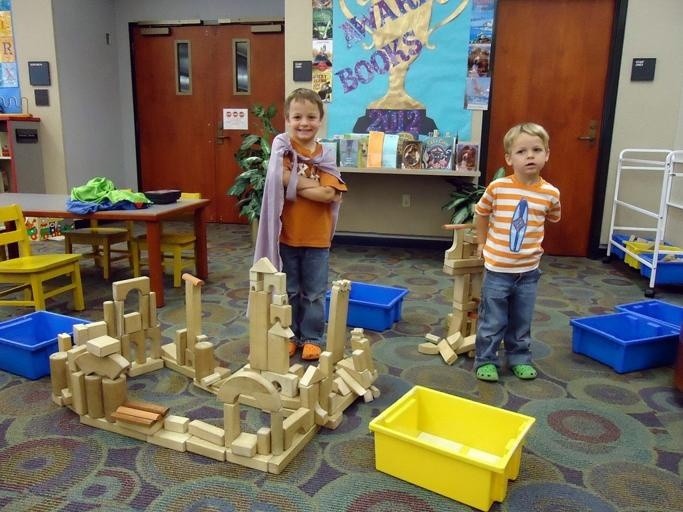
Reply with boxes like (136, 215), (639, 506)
(0, 114), (46, 193)
(327, 167), (481, 194)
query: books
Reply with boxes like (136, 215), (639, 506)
(321, 131), (480, 173)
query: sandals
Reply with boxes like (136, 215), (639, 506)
(510, 363), (537, 379)
(476, 365), (500, 383)
(299, 345), (322, 360)
(288, 342), (297, 356)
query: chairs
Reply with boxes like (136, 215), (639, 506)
(132, 193), (201, 288)
(61, 189), (134, 279)
(1, 203), (85, 313)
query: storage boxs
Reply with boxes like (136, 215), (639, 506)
(0, 311), (94, 381)
(641, 252), (683, 283)
(622, 239), (682, 269)
(570, 312), (679, 374)
(611, 233), (673, 259)
(326, 281), (410, 332)
(368, 385), (536, 512)
(614, 300), (683, 332)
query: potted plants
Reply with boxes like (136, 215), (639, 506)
(227, 101), (282, 249)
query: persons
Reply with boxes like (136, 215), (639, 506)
(473, 123), (561, 381)
(246, 88), (347, 361)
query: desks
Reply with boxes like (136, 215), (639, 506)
(0, 193), (212, 308)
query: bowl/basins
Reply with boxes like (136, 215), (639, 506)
(145, 190), (181, 203)
